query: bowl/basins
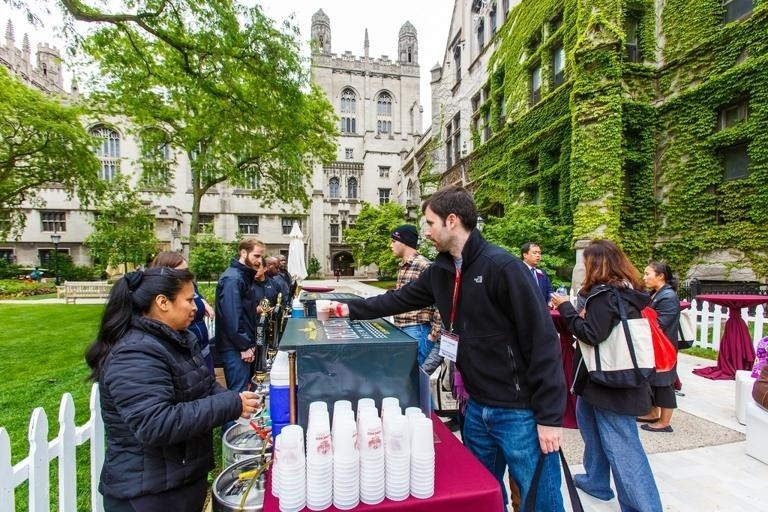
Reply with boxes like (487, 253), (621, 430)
(222, 415), (273, 469)
(211, 455), (272, 512)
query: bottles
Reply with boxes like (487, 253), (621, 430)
(556, 284), (567, 296)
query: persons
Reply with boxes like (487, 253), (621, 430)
(277, 254), (295, 302)
(519, 241), (549, 304)
(420, 336), (443, 375)
(390, 225), (443, 366)
(335, 268), (341, 282)
(278, 258), (290, 283)
(548, 241), (664, 512)
(269, 259), (287, 307)
(254, 256), (285, 314)
(321, 185), (567, 512)
(84, 266), (262, 512)
(636, 261), (680, 432)
(266, 257), (289, 302)
(152, 250), (217, 377)
(663, 264), (685, 397)
(215, 237), (266, 392)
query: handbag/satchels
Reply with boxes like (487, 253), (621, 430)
(677, 301), (695, 349)
(577, 285), (656, 388)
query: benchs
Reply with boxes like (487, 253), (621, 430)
(64, 280), (117, 304)
(56, 280), (108, 300)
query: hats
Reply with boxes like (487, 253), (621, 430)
(390, 225), (419, 249)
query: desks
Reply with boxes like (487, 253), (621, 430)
(696, 294), (764, 381)
(548, 309), (579, 429)
(260, 410), (504, 512)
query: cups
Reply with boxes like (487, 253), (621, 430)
(306, 401), (332, 510)
(316, 300), (330, 320)
(406, 406), (435, 500)
(272, 424), (306, 511)
(382, 396), (410, 501)
(332, 401), (359, 509)
(356, 398), (385, 506)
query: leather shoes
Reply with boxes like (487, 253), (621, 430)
(635, 416), (659, 423)
(640, 423), (675, 433)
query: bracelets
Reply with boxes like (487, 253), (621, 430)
(336, 302), (344, 318)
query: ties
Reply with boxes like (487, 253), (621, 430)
(530, 267), (539, 285)
